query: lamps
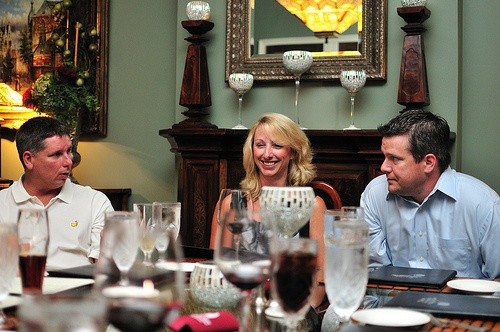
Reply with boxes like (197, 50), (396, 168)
(276, 0), (362, 44)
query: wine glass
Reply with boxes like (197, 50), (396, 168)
(153, 201), (184, 266)
(133, 201), (162, 266)
(217, 188), (255, 266)
(102, 208), (143, 290)
(267, 234), (319, 332)
(321, 203), (371, 332)
(213, 197), (275, 332)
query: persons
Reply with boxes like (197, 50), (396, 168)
(358, 109), (499, 280)
(208, 112), (329, 304)
(0, 116), (116, 272)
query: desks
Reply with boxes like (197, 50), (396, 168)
(0, 262), (500, 332)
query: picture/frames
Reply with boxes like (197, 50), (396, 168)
(0, 0), (108, 137)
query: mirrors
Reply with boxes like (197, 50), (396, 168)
(226, 0), (387, 83)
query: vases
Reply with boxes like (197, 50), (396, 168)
(69, 136), (82, 186)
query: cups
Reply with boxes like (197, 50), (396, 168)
(96, 215), (183, 332)
(16, 207), (50, 302)
(13, 298), (112, 331)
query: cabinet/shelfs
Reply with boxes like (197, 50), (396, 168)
(160, 128), (455, 263)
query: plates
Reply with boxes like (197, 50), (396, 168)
(155, 259), (199, 273)
(352, 306), (431, 328)
(447, 278), (500, 295)
